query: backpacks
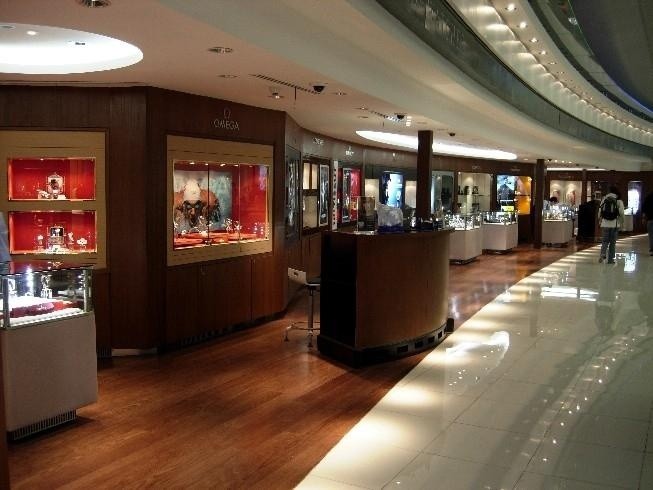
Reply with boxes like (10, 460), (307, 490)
(600, 196), (620, 221)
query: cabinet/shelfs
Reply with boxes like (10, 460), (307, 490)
(449, 212), (483, 265)
(0, 262), (99, 440)
(542, 215), (576, 248)
(616, 214), (634, 234)
(166, 134), (274, 268)
(0, 130), (106, 271)
(483, 211), (519, 255)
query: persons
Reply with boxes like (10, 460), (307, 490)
(472, 186), (478, 194)
(174, 180), (219, 228)
(498, 177), (513, 205)
(640, 192), (653, 256)
(598, 185), (625, 264)
(588, 192), (600, 242)
(49, 178), (63, 195)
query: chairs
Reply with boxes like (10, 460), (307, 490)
(284, 266), (320, 347)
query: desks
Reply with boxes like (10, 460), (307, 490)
(316, 220), (454, 370)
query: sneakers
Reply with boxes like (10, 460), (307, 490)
(608, 258), (615, 264)
(599, 256), (606, 263)
